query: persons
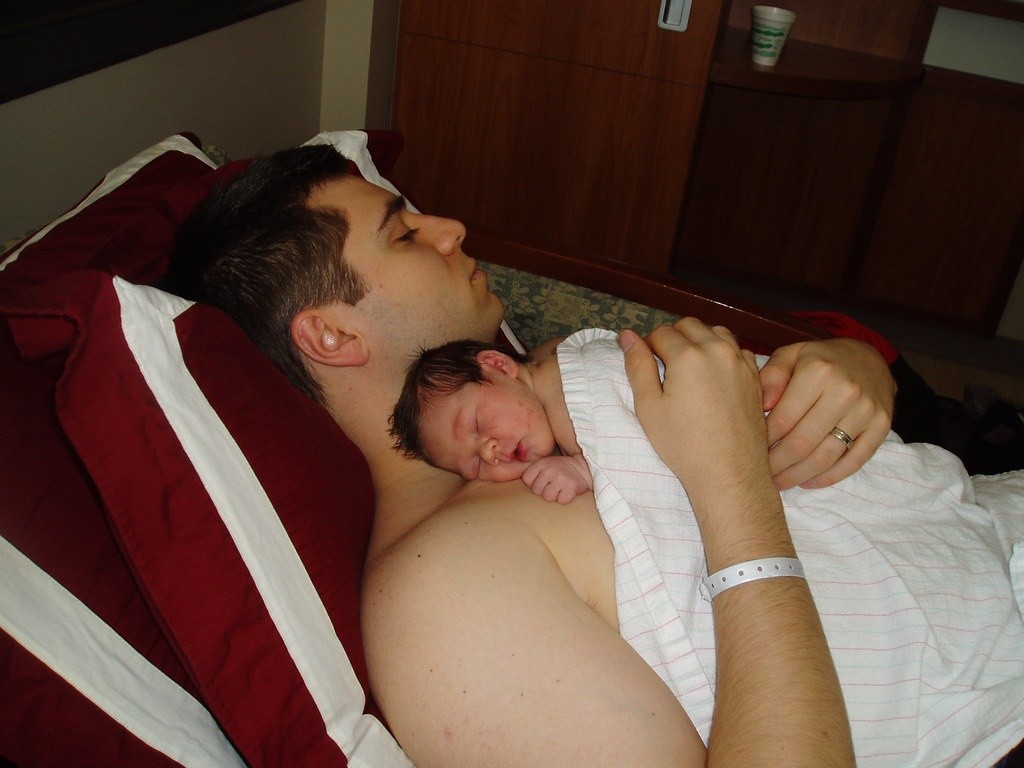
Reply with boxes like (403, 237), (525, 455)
(166, 145), (1023, 768)
(387, 334), (903, 506)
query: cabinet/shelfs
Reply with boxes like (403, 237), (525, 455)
(392, 0), (939, 305)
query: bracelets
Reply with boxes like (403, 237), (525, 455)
(699, 557), (807, 602)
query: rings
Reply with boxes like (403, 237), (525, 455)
(830, 426), (854, 448)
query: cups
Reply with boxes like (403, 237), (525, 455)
(752, 6), (796, 66)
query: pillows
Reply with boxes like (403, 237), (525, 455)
(1, 129), (529, 768)
